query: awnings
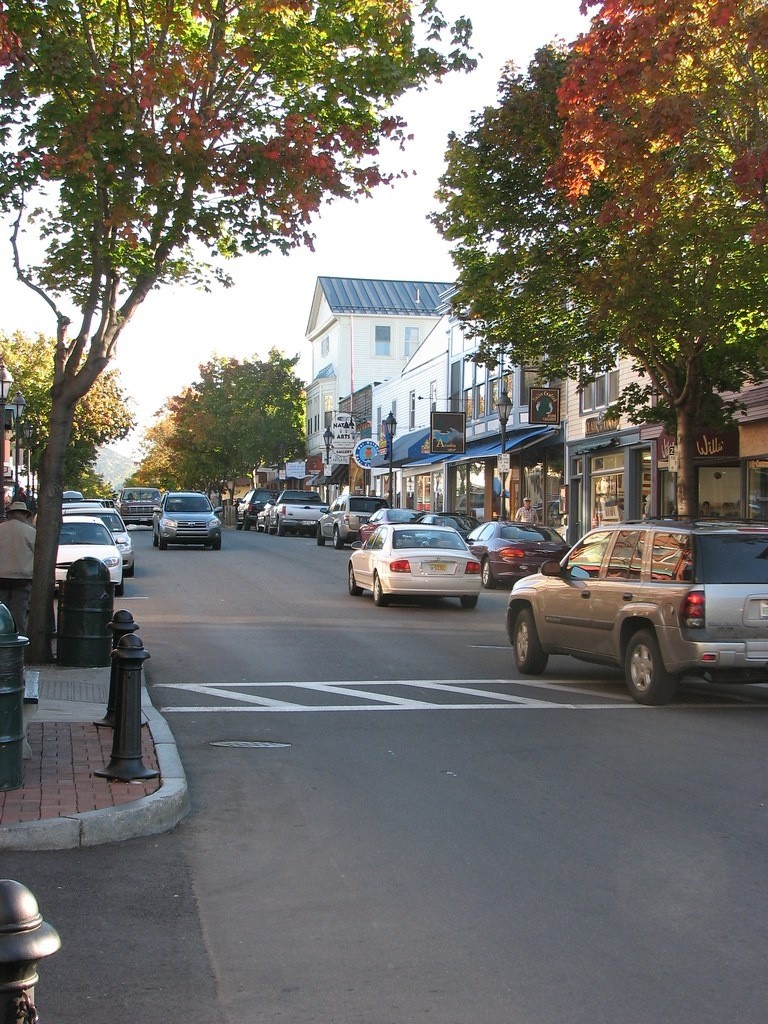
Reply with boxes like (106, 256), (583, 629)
(265, 476), (292, 486)
(402, 426), (560, 467)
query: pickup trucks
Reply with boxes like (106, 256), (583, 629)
(266, 489), (331, 537)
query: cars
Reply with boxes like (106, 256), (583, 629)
(413, 511), (482, 544)
(256, 498), (277, 534)
(53, 516), (126, 597)
(456, 521), (574, 589)
(58, 508), (135, 578)
(62, 490), (120, 519)
(356, 508), (423, 545)
(346, 524), (482, 609)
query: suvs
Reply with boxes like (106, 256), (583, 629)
(316, 493), (389, 550)
(117, 488), (162, 529)
(505, 515), (768, 707)
(151, 489), (223, 550)
(236, 488), (279, 532)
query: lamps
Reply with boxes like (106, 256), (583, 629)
(343, 414), (367, 429)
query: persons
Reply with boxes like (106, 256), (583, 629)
(0, 502), (36, 636)
(149, 491), (160, 500)
(192, 500), (206, 511)
(127, 493), (136, 500)
(2, 485), (40, 519)
(514, 498), (539, 524)
(167, 502), (179, 511)
(671, 502), (741, 517)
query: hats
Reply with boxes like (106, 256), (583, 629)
(6, 501), (33, 517)
(523, 497), (530, 500)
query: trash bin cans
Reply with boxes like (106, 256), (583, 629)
(54, 557), (115, 668)
(0, 603), (29, 792)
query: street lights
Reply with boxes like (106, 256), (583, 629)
(322, 427), (334, 507)
(22, 420), (35, 498)
(496, 387), (515, 525)
(0, 363), (15, 524)
(384, 411), (398, 510)
(10, 390), (26, 504)
